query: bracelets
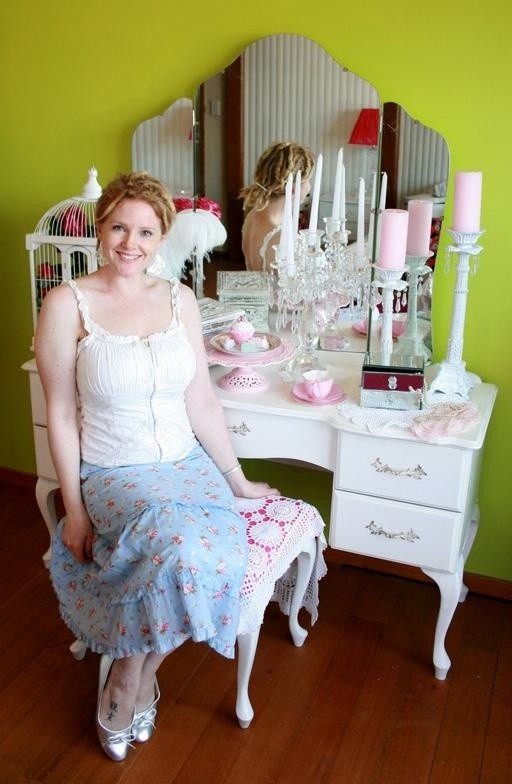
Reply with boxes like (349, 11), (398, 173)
(224, 464), (242, 477)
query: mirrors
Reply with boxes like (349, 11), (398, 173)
(130, 30), (451, 366)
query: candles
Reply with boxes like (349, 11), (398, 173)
(405, 197), (435, 257)
(454, 168), (485, 234)
(294, 144), (388, 259)
(272, 166), (366, 270)
(376, 205), (406, 268)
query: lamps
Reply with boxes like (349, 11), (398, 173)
(343, 106), (380, 148)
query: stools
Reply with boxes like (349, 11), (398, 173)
(67, 476), (330, 731)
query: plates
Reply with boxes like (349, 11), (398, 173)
(290, 380), (345, 403)
(209, 331), (283, 357)
(352, 318), (407, 339)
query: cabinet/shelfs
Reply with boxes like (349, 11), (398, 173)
(20, 339), (498, 683)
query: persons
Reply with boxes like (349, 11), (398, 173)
(237, 141), (316, 272)
(35, 171), (281, 762)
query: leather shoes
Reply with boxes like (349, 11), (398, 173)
(96, 651), (136, 764)
(130, 675), (162, 744)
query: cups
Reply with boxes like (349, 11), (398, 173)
(302, 369), (335, 400)
